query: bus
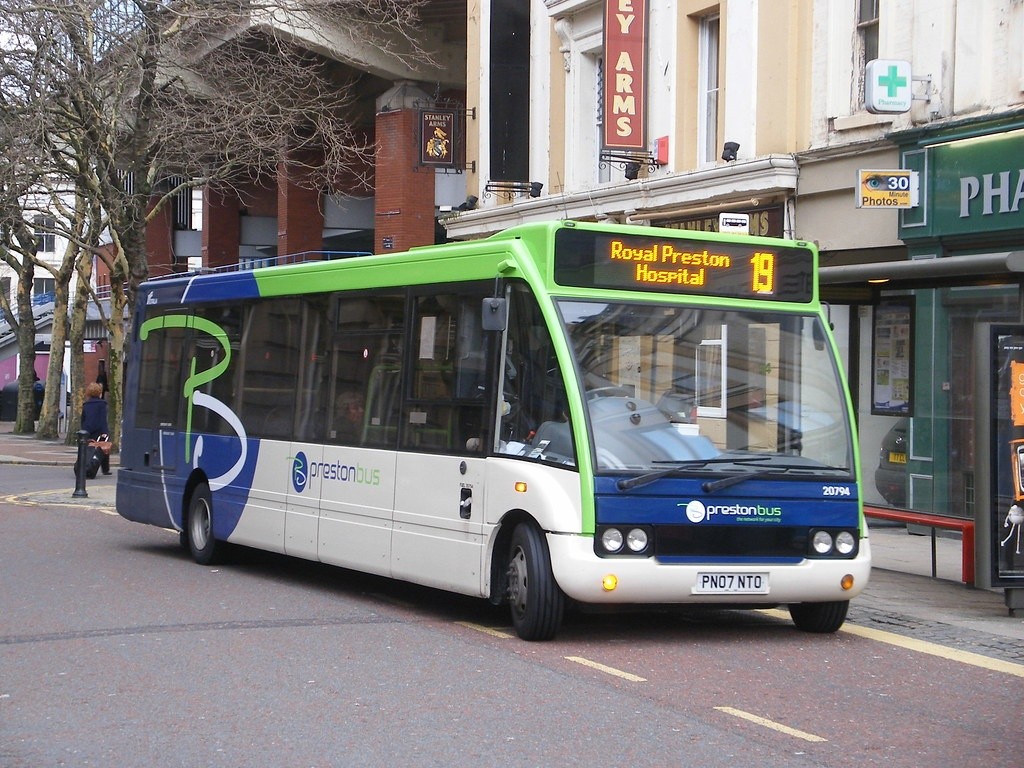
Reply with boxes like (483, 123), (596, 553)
(115, 220), (873, 641)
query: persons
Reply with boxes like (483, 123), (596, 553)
(81, 383), (113, 475)
(463, 379), (536, 452)
(334, 390), (365, 445)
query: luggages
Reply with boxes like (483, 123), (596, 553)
(73, 435), (107, 479)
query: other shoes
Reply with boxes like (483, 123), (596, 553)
(103, 473), (113, 476)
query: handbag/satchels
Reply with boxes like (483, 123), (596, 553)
(88, 435), (113, 454)
